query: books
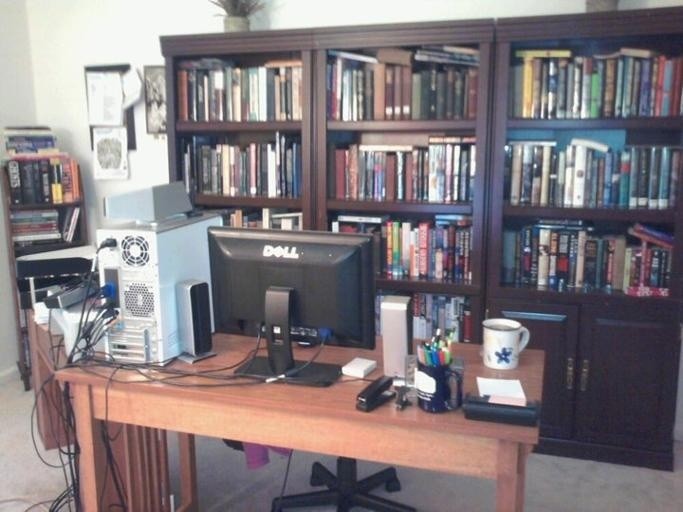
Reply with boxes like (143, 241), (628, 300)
(475, 373), (529, 409)
(0, 123), (84, 250)
(501, 48), (682, 297)
(175, 55), (302, 233)
(412, 292), (473, 346)
(327, 45), (480, 282)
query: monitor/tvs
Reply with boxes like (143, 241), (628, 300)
(206, 226), (376, 388)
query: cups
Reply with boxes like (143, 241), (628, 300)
(481, 318), (530, 371)
(413, 360), (462, 412)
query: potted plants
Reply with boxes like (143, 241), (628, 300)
(209, 0), (266, 32)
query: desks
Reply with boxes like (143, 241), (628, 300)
(51, 323), (546, 511)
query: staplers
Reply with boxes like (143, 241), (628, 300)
(357, 376), (396, 411)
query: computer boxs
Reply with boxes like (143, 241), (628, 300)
(95, 213), (227, 368)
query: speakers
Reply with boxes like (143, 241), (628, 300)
(175, 279), (215, 365)
(380, 293), (414, 376)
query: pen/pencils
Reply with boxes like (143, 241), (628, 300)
(417, 328), (454, 367)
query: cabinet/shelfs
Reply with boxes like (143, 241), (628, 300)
(0, 161), (86, 390)
